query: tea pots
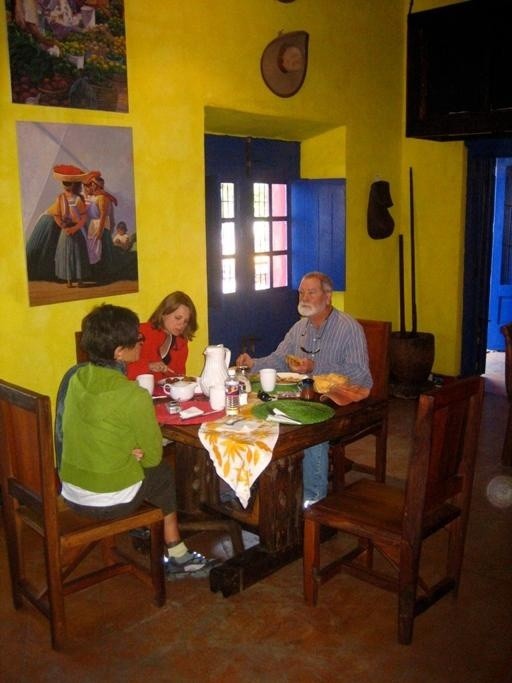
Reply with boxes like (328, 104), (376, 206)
(200, 344), (231, 396)
(163, 381), (199, 402)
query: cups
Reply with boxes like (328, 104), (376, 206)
(137, 374), (154, 395)
(210, 385), (224, 410)
(260, 369), (276, 392)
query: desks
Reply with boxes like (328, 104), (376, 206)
(133, 380), (389, 598)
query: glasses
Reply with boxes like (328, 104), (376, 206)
(125, 332), (145, 345)
(300, 334), (320, 354)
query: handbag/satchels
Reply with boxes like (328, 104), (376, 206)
(368, 191), (394, 239)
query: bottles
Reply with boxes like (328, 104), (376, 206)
(224, 370), (239, 416)
(239, 384), (248, 406)
(301, 379), (315, 400)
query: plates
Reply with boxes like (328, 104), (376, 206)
(276, 372), (308, 386)
(158, 376), (201, 388)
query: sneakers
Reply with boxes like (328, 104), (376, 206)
(163, 549), (223, 581)
(128, 526), (150, 539)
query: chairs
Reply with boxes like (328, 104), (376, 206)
(75, 328), (144, 369)
(498, 320), (512, 476)
(299, 318), (398, 487)
(1, 382), (170, 649)
(297, 375), (488, 646)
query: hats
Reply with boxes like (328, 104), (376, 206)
(319, 381), (370, 406)
(260, 31), (309, 96)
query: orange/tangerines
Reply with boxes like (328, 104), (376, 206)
(60, 16), (126, 70)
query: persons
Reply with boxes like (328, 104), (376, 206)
(14, 0), (112, 57)
(49, 163), (132, 288)
(224, 271), (372, 514)
(59, 302), (225, 581)
(126, 290), (198, 382)
